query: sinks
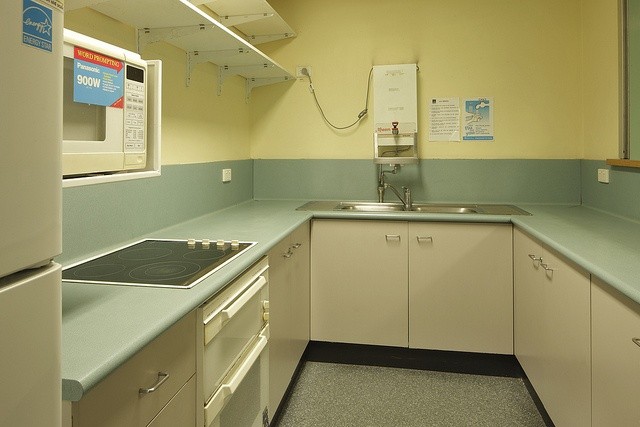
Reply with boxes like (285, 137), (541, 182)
(341, 202), (407, 213)
(411, 205), (478, 214)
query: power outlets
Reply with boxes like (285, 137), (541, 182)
(295, 65), (313, 78)
(222, 169), (232, 183)
(598, 169), (609, 185)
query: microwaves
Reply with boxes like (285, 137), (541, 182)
(64, 27), (147, 178)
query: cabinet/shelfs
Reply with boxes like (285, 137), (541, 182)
(267, 220), (311, 423)
(62, 59), (164, 189)
(309, 218), (512, 379)
(187, 0), (298, 46)
(590, 273), (639, 427)
(64, 307), (200, 427)
(512, 219), (590, 427)
(64, 0), (296, 104)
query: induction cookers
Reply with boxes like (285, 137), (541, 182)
(62, 237), (258, 289)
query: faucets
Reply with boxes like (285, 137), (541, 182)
(384, 184), (412, 209)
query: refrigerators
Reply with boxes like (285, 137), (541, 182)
(1, 0), (64, 427)
